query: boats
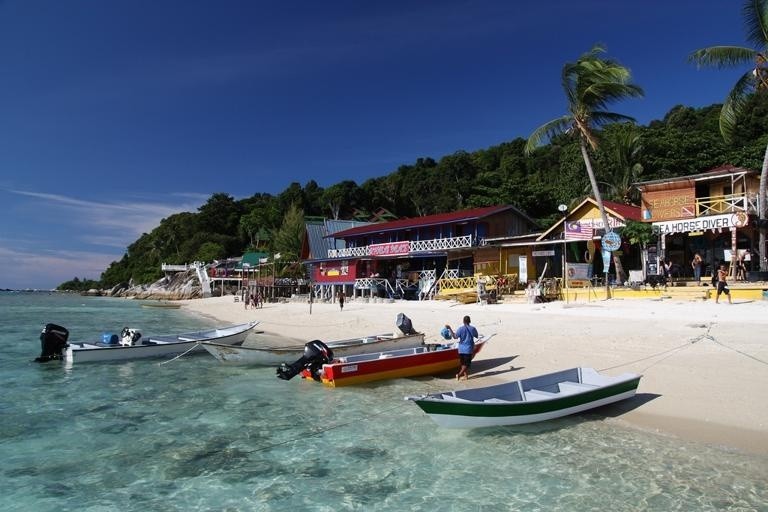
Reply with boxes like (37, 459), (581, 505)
(32, 319), (262, 366)
(138, 302), (181, 309)
(197, 313), (425, 367)
(404, 364), (644, 431)
(276, 332), (499, 388)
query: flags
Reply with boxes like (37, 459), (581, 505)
(260, 258), (268, 263)
(274, 252), (281, 260)
(242, 263), (250, 268)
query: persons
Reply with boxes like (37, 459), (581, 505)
(659, 249), (750, 305)
(242, 288), (262, 309)
(337, 288), (347, 311)
(444, 315), (478, 381)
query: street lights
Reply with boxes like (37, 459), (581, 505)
(557, 203), (568, 288)
